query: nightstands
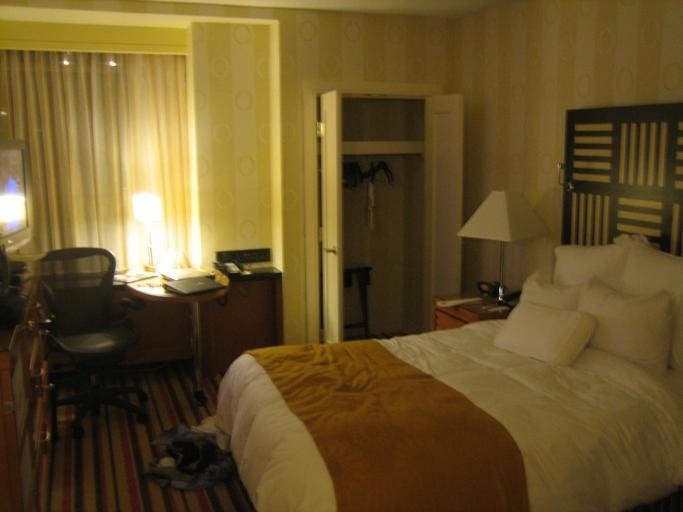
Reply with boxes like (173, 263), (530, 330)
(431, 289), (520, 332)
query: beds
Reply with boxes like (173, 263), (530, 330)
(213, 319), (682, 511)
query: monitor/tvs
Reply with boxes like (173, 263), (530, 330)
(0, 138), (33, 255)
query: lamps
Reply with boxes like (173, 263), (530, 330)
(455, 187), (550, 311)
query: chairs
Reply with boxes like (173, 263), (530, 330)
(38, 245), (148, 439)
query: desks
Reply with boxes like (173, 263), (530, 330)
(33, 264), (284, 405)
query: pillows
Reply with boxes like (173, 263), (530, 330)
(493, 233), (682, 374)
